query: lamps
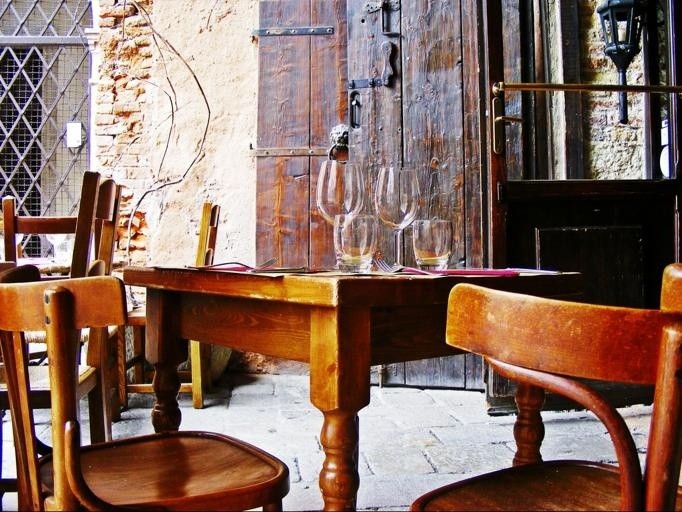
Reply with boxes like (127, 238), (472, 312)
(597, 1), (650, 124)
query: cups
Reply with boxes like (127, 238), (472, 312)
(411, 219), (453, 269)
(335, 214), (378, 274)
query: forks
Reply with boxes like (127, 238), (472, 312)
(185, 257), (278, 270)
(374, 259), (449, 276)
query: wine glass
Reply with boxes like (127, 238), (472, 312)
(374, 166), (419, 272)
(315, 161), (366, 254)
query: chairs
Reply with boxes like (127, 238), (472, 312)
(42, 286), (290, 511)
(407, 259), (681, 512)
(3, 273), (124, 508)
(0, 172), (120, 418)
(127, 203), (222, 407)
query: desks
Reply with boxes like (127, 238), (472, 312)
(121, 263), (580, 509)
